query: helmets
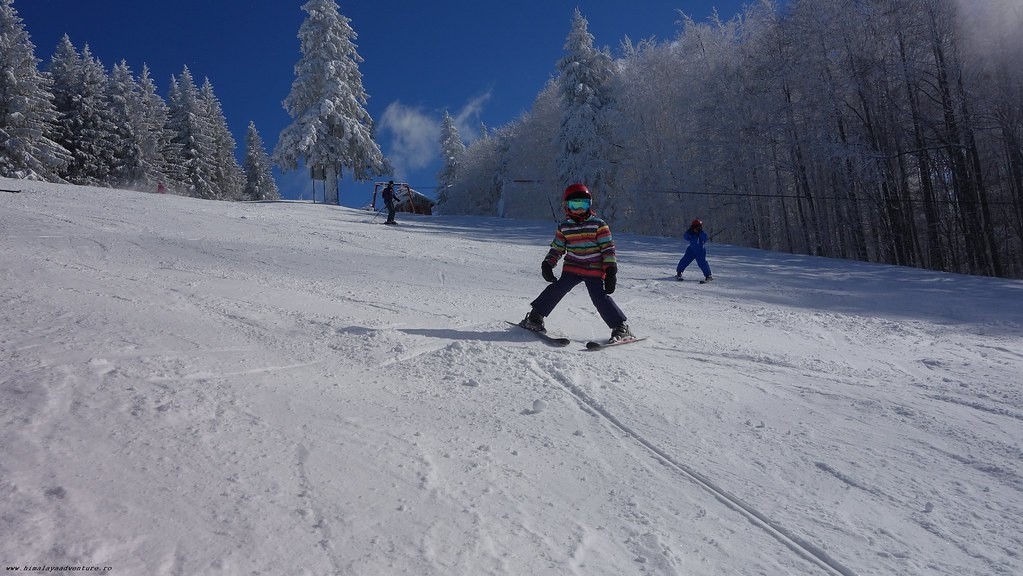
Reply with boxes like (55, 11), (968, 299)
(562, 183), (591, 220)
(691, 218), (702, 231)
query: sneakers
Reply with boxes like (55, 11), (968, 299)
(607, 320), (636, 343)
(518, 309), (546, 334)
(676, 272), (683, 280)
(699, 274), (712, 284)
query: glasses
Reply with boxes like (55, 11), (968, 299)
(562, 197), (591, 210)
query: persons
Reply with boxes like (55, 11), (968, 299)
(382, 180), (402, 225)
(674, 216), (718, 283)
(522, 179), (637, 346)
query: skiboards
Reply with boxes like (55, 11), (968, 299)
(504, 318), (646, 348)
(677, 275), (714, 283)
